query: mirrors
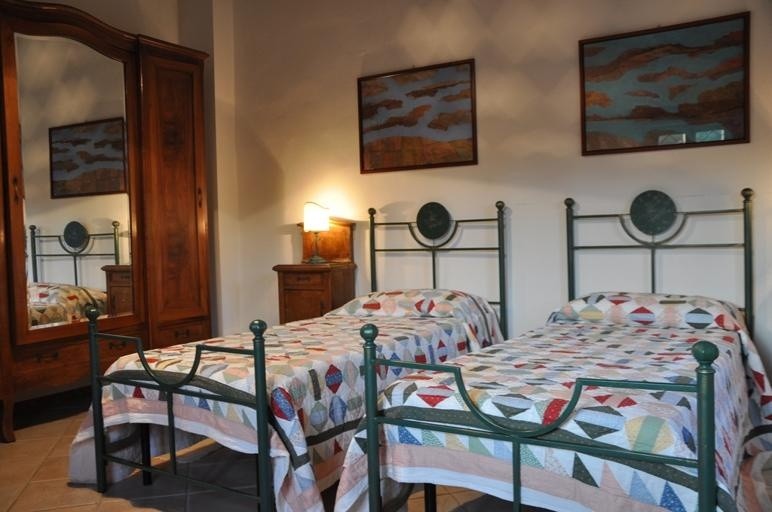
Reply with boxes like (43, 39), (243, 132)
(10, 32), (145, 347)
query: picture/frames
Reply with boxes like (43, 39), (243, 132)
(46, 114), (123, 199)
(578, 10), (752, 155)
(356, 57), (480, 174)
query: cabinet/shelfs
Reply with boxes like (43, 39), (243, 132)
(0, 1), (214, 443)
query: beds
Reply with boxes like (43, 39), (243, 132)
(360, 187), (755, 511)
(28, 222), (119, 326)
(86, 201), (507, 512)
